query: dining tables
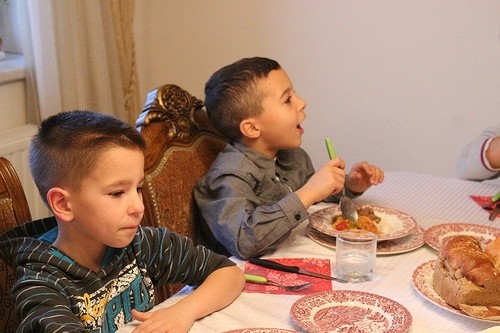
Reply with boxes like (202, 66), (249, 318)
(115, 168), (500, 331)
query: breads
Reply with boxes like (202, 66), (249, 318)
(430, 235), (500, 321)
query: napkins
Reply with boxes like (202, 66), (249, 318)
(241, 257), (332, 295)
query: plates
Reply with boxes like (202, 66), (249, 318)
(305, 222), (426, 255)
(410, 259), (500, 324)
(289, 290), (413, 333)
(222, 328), (297, 333)
(422, 223), (500, 251)
(309, 204), (417, 243)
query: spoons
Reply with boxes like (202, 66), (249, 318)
(325, 138), (358, 223)
(245, 274), (314, 291)
(482, 192), (500, 210)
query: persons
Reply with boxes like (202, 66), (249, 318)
(456, 116), (500, 181)
(194, 57), (383, 260)
(0, 111), (245, 333)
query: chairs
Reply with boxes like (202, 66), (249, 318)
(0, 154), (33, 331)
(134, 83), (231, 244)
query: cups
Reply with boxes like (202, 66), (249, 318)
(334, 229), (378, 283)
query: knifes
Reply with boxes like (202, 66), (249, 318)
(248, 257), (348, 283)
(489, 202), (500, 221)
